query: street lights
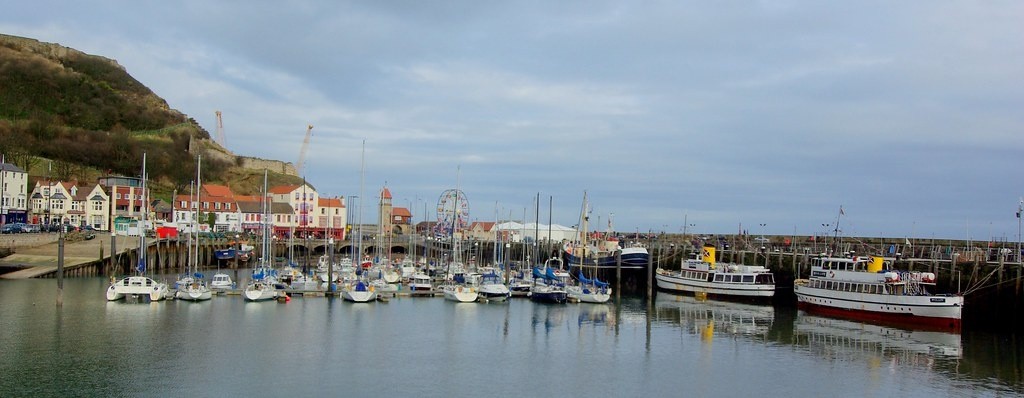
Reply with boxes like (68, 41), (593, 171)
(1018, 196), (1024, 263)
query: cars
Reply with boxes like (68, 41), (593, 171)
(3, 223), (95, 241)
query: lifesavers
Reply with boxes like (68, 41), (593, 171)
(829, 270), (835, 278)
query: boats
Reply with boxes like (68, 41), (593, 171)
(560, 217), (647, 269)
(654, 253), (774, 298)
(793, 250), (961, 335)
(653, 293), (774, 336)
(792, 312), (963, 370)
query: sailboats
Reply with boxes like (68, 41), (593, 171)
(92, 150), (613, 306)
